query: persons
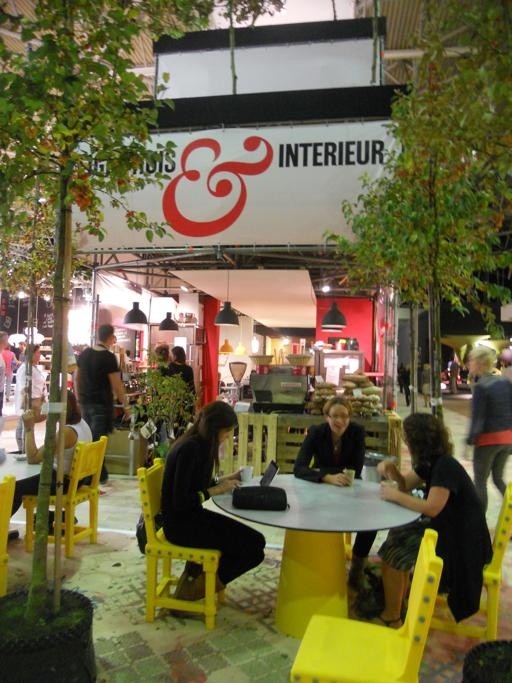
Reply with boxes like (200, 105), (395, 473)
(462, 344), (511, 514)
(294, 398), (377, 595)
(10, 389), (94, 517)
(160, 400), (265, 617)
(369, 412), (492, 628)
(397, 362), (451, 408)
(0, 323), (197, 486)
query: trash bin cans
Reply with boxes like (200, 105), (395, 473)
(360, 450), (386, 483)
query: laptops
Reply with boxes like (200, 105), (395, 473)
(233, 460), (279, 492)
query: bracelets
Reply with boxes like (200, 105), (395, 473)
(198, 491), (205, 503)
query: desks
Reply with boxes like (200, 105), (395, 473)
(1, 451), (48, 494)
(212, 473), (427, 641)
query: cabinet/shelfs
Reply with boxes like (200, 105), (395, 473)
(144, 308), (202, 396)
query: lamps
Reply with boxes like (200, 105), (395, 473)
(219, 327), (235, 355)
(158, 267), (180, 333)
(122, 257), (152, 327)
(214, 257), (242, 327)
(320, 325), (343, 333)
(32, 293), (45, 345)
(318, 265), (350, 327)
(9, 295), (26, 347)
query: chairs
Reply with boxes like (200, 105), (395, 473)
(419, 483), (512, 644)
(136, 456), (220, 628)
(28, 432), (112, 554)
(287, 526), (443, 682)
(0, 476), (24, 596)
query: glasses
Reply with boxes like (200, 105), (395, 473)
(326, 412), (350, 421)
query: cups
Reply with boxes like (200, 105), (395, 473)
(342, 468), (357, 483)
(238, 464), (254, 484)
(380, 479), (398, 487)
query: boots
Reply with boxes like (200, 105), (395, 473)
(169, 571), (225, 617)
(349, 553), (373, 593)
